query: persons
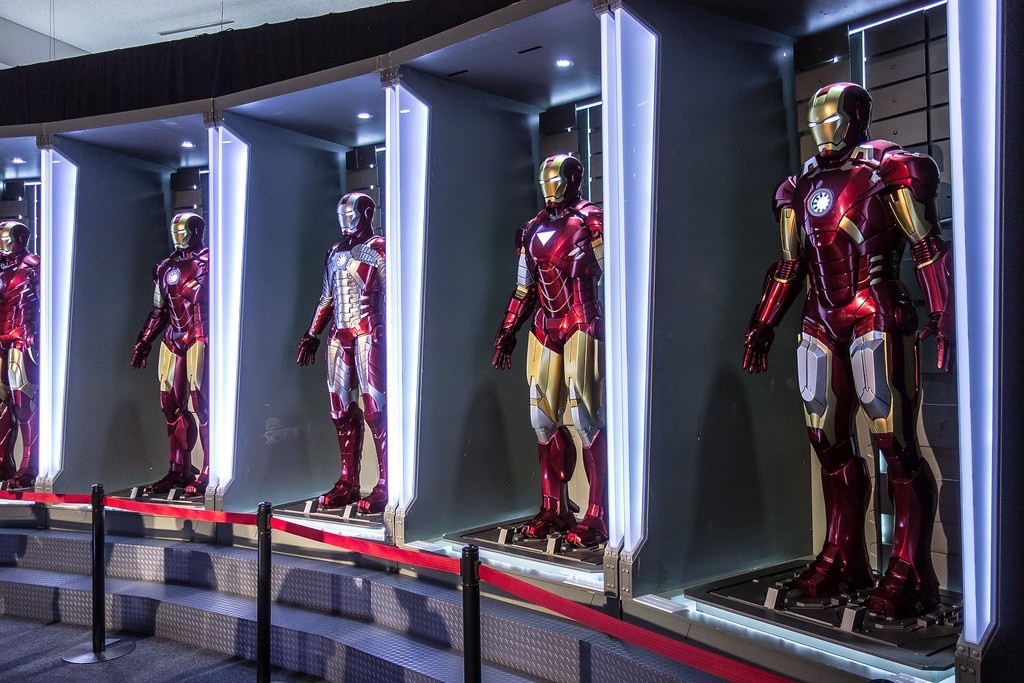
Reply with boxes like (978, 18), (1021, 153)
(130, 212), (209, 496)
(492, 154), (608, 546)
(295, 193), (388, 514)
(742, 83), (954, 620)
(0, 221), (41, 488)
